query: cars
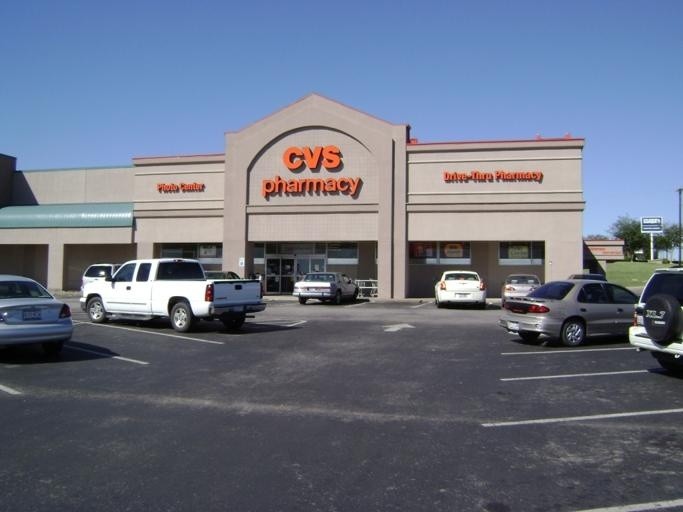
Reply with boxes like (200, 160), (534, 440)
(496, 279), (639, 347)
(431, 270), (486, 307)
(0, 274), (73, 348)
(80, 263), (121, 291)
(632, 254), (646, 262)
(292, 272), (358, 305)
(500, 274), (543, 308)
(204, 270), (240, 279)
(567, 273), (607, 282)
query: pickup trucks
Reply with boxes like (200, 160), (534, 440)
(78, 257), (266, 333)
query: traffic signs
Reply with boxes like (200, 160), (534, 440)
(642, 218), (662, 232)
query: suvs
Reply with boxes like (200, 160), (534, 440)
(627, 266), (682, 372)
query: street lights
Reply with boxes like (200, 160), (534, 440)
(674, 185), (683, 267)
